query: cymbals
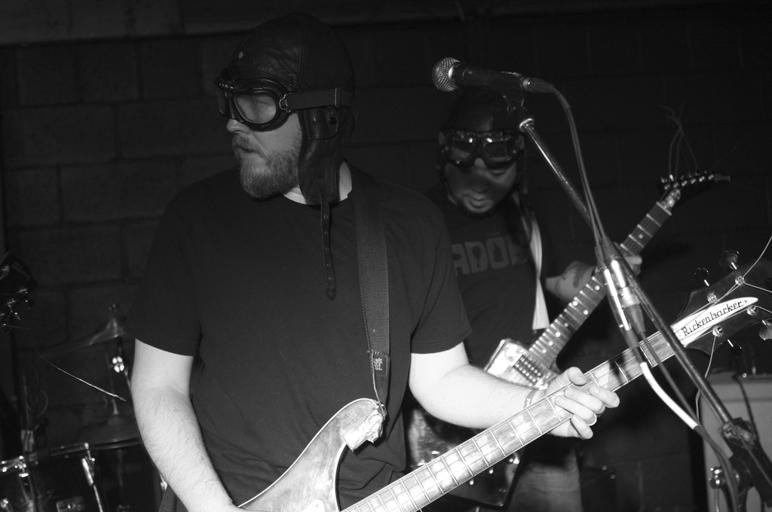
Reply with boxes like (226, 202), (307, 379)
(35, 415), (142, 462)
(39, 318), (125, 357)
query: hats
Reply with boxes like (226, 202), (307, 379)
(225, 12), (356, 142)
(444, 87), (525, 134)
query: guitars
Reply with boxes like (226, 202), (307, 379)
(408, 172), (733, 509)
(237, 240), (771, 512)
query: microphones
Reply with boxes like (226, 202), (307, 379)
(430, 54), (528, 100)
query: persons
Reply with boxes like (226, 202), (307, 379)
(388, 82), (645, 512)
(116, 30), (624, 512)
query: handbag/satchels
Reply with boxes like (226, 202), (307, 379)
(404, 402), (526, 509)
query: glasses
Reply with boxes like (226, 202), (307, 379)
(215, 66), (352, 134)
(436, 127), (523, 170)
